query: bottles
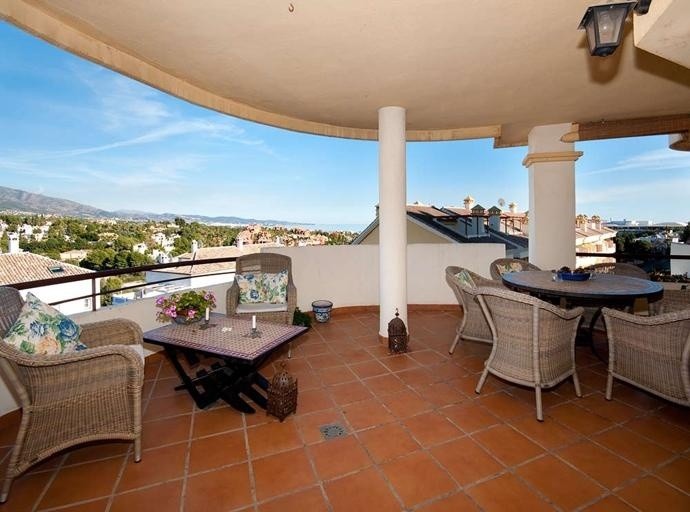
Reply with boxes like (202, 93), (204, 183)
(558, 270), (563, 283)
(552, 269), (558, 281)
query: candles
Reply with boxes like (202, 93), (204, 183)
(206, 307), (209, 320)
(251, 316), (256, 329)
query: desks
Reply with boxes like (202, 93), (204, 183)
(143, 312), (309, 414)
(501, 272), (664, 365)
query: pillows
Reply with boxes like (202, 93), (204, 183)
(497, 263), (522, 277)
(1, 291), (83, 356)
(236, 270), (289, 304)
(451, 269), (476, 289)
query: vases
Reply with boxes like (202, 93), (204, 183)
(170, 315), (202, 324)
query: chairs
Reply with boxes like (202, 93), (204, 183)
(446, 266), (510, 355)
(490, 258), (541, 281)
(601, 288), (690, 407)
(576, 262), (649, 331)
(227, 252), (297, 358)
(0, 287), (145, 505)
(474, 286), (585, 422)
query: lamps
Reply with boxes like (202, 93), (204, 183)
(577, 1), (637, 58)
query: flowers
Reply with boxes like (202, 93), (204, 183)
(154, 291), (218, 323)
